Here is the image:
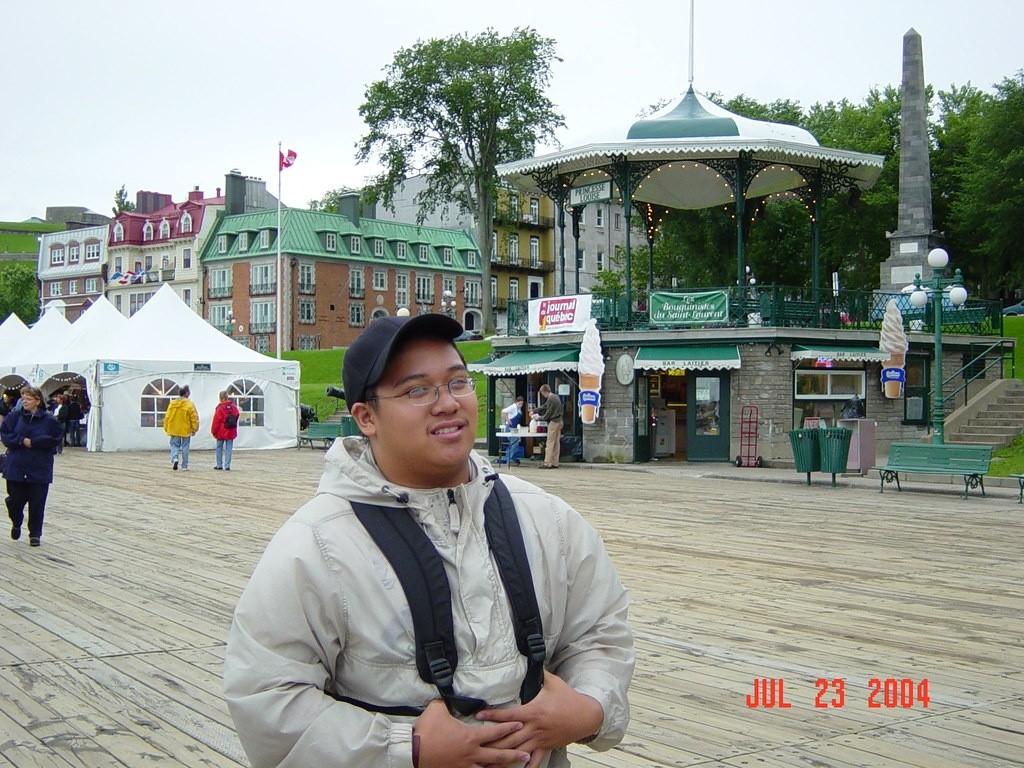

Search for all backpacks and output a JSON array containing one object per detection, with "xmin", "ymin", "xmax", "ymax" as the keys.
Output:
[{"xmin": 222, "ymin": 405, "xmax": 237, "ymax": 428}]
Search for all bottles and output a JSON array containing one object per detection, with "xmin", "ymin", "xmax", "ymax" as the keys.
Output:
[
  {"xmin": 529, "ymin": 419, "xmax": 537, "ymax": 433},
  {"xmin": 517, "ymin": 423, "xmax": 521, "ymax": 435}
]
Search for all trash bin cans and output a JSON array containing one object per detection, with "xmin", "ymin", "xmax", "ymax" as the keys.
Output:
[
  {"xmin": 340, "ymin": 415, "xmax": 360, "ymax": 436},
  {"xmin": 788, "ymin": 428, "xmax": 854, "ymax": 474}
]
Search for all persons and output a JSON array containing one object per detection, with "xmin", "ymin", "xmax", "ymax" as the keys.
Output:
[
  {"xmin": 670, "ymin": 385, "xmax": 681, "ymax": 402},
  {"xmin": 529, "ymin": 385, "xmax": 563, "ymax": 469},
  {"xmin": 0, "ymin": 388, "xmax": 30, "ymax": 440},
  {"xmin": 0, "ymin": 388, "xmax": 63, "ymax": 546},
  {"xmin": 502, "ymin": 396, "xmax": 524, "ymax": 466},
  {"xmin": 163, "ymin": 385, "xmax": 199, "ymax": 471},
  {"xmin": 47, "ymin": 391, "xmax": 82, "ymax": 454},
  {"xmin": 212, "ymin": 391, "xmax": 239, "ymax": 470},
  {"xmin": 223, "ymin": 314, "xmax": 636, "ymax": 768}
]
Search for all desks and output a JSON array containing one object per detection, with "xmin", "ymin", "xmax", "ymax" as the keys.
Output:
[{"xmin": 496, "ymin": 432, "xmax": 565, "ymax": 470}]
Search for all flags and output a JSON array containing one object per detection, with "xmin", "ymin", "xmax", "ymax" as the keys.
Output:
[
  {"xmin": 111, "ymin": 269, "xmax": 145, "ymax": 284},
  {"xmin": 280, "ymin": 149, "xmax": 297, "ymax": 171}
]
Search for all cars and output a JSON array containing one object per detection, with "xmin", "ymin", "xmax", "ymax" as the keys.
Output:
[{"xmin": 1002, "ymin": 299, "xmax": 1024, "ymax": 317}]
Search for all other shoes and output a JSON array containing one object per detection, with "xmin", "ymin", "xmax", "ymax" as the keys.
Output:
[
  {"xmin": 30, "ymin": 537, "xmax": 40, "ymax": 546},
  {"xmin": 552, "ymin": 466, "xmax": 558, "ymax": 469},
  {"xmin": 183, "ymin": 469, "xmax": 188, "ymax": 470},
  {"xmin": 173, "ymin": 460, "xmax": 179, "ymax": 470},
  {"xmin": 11, "ymin": 526, "xmax": 21, "ymax": 539},
  {"xmin": 214, "ymin": 467, "xmax": 222, "ymax": 470},
  {"xmin": 538, "ymin": 465, "xmax": 552, "ymax": 468},
  {"xmin": 226, "ymin": 468, "xmax": 230, "ymax": 470}
]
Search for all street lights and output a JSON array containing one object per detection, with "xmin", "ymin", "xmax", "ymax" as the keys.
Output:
[
  {"xmin": 909, "ymin": 247, "xmax": 968, "ymax": 445},
  {"xmin": 290, "ymin": 257, "xmax": 297, "ymax": 351},
  {"xmin": 225, "ymin": 310, "xmax": 236, "ymax": 336},
  {"xmin": 440, "ymin": 290, "xmax": 457, "ymax": 318}
]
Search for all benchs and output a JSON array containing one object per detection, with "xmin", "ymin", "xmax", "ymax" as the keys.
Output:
[
  {"xmin": 597, "ymin": 298, "xmax": 815, "ymax": 331},
  {"xmin": 873, "ymin": 305, "xmax": 986, "ymax": 335},
  {"xmin": 870, "ymin": 441, "xmax": 994, "ymax": 500},
  {"xmin": 298, "ymin": 421, "xmax": 342, "ymax": 452},
  {"xmin": 1009, "ymin": 471, "xmax": 1024, "ymax": 503}
]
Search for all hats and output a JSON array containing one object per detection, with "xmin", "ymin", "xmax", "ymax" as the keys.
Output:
[{"xmin": 342, "ymin": 313, "xmax": 464, "ymax": 413}]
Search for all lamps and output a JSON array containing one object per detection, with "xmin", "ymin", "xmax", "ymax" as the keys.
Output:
[
  {"xmin": 605, "ymin": 351, "xmax": 612, "ymax": 363},
  {"xmin": 765, "ymin": 343, "xmax": 772, "ymax": 357},
  {"xmin": 774, "ymin": 345, "xmax": 785, "ymax": 357}
]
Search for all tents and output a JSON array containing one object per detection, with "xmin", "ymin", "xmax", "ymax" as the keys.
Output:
[{"xmin": 0, "ymin": 283, "xmax": 301, "ymax": 452}]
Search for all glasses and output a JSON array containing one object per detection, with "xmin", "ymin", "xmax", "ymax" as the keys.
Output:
[{"xmin": 365, "ymin": 377, "xmax": 477, "ymax": 406}]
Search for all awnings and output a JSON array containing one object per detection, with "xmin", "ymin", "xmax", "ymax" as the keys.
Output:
[
  {"xmin": 484, "ymin": 348, "xmax": 579, "ymax": 394},
  {"xmin": 790, "ymin": 345, "xmax": 891, "ymax": 372},
  {"xmin": 467, "ymin": 357, "xmax": 492, "ymax": 373},
  {"xmin": 634, "ymin": 347, "xmax": 741, "ymax": 370}
]
